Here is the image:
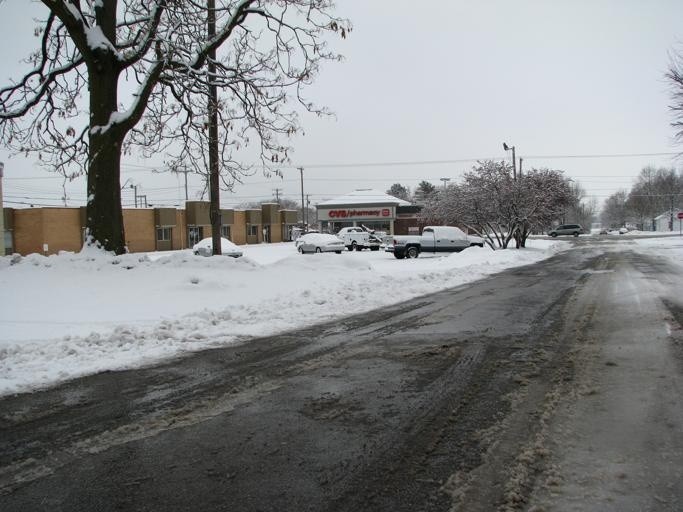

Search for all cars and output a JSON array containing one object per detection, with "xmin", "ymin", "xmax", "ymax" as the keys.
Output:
[
  {"xmin": 619, "ymin": 227, "xmax": 628, "ymax": 233},
  {"xmin": 294, "ymin": 233, "xmax": 345, "ymax": 254},
  {"xmin": 192, "ymin": 236, "xmax": 242, "ymax": 259}
]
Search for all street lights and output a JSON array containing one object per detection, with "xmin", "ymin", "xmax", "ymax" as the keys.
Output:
[
  {"xmin": 503, "ymin": 143, "xmax": 516, "ymax": 182},
  {"xmin": 440, "ymin": 178, "xmax": 450, "ymax": 188}
]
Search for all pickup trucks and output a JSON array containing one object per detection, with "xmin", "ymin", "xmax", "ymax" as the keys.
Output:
[
  {"xmin": 334, "ymin": 226, "xmax": 387, "ymax": 251},
  {"xmin": 384, "ymin": 225, "xmax": 485, "ymax": 258}
]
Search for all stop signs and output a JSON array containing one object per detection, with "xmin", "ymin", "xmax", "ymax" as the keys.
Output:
[{"xmin": 677, "ymin": 213, "xmax": 683, "ymax": 219}]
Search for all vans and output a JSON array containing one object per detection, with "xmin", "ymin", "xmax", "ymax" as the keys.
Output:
[{"xmin": 548, "ymin": 223, "xmax": 584, "ymax": 237}]
